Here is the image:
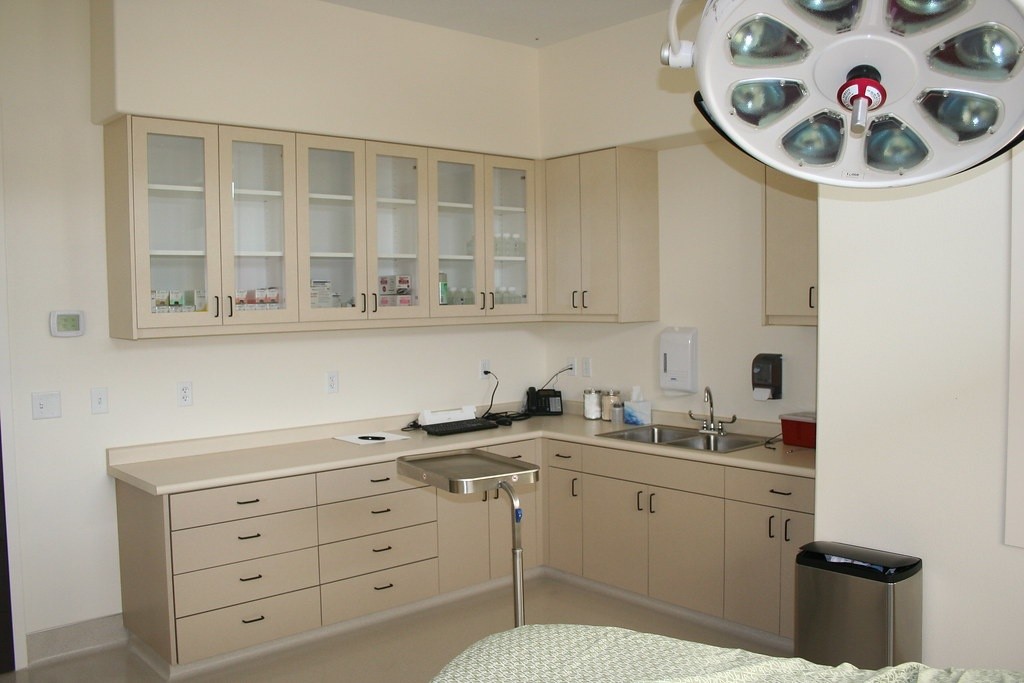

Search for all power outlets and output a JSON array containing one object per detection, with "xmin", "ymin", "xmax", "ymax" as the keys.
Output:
[
  {"xmin": 326, "ymin": 370, "xmax": 338, "ymax": 394},
  {"xmin": 479, "ymin": 358, "xmax": 491, "ymax": 380},
  {"xmin": 177, "ymin": 381, "xmax": 192, "ymax": 408},
  {"xmin": 566, "ymin": 356, "xmax": 576, "ymax": 377}
]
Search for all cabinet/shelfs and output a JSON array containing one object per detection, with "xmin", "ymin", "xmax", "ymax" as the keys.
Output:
[
  {"xmin": 426, "ymin": 147, "xmax": 543, "ymax": 326},
  {"xmin": 104, "ymin": 114, "xmax": 298, "ymax": 339},
  {"xmin": 546, "ymin": 143, "xmax": 660, "ymax": 325},
  {"xmin": 295, "ymin": 132, "xmax": 429, "ymax": 333},
  {"xmin": 114, "ymin": 437, "xmax": 815, "ymax": 679},
  {"xmin": 760, "ymin": 162, "xmax": 818, "ymax": 329}
]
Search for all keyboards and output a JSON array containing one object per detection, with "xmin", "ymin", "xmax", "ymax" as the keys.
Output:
[{"xmin": 421, "ymin": 418, "xmax": 498, "ymax": 436}]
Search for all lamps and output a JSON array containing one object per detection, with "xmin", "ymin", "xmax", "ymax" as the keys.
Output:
[{"xmin": 659, "ymin": 0, "xmax": 1024, "ymax": 189}]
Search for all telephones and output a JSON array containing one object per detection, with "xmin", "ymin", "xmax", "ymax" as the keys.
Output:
[{"xmin": 526, "ymin": 386, "xmax": 563, "ymax": 415}]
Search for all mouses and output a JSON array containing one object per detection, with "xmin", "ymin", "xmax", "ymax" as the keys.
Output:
[{"xmin": 496, "ymin": 418, "xmax": 512, "ymax": 426}]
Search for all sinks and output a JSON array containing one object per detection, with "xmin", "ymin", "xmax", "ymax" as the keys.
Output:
[
  {"xmin": 594, "ymin": 424, "xmax": 700, "ymax": 445},
  {"xmin": 666, "ymin": 434, "xmax": 782, "ymax": 454}
]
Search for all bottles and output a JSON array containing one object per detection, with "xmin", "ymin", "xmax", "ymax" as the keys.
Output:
[
  {"xmin": 447, "ymin": 287, "xmax": 522, "ymax": 306},
  {"xmin": 612, "ymin": 402, "xmax": 624, "ymax": 425},
  {"xmin": 466, "ymin": 233, "xmax": 523, "ymax": 257}
]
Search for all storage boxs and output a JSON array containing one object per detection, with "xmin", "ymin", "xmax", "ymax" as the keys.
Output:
[
  {"xmin": 150, "ymin": 290, "xmax": 208, "ymax": 313},
  {"xmin": 378, "ymin": 275, "xmax": 411, "ymax": 306},
  {"xmin": 235, "ymin": 288, "xmax": 281, "ymax": 311},
  {"xmin": 310, "ymin": 280, "xmax": 342, "ymax": 307}
]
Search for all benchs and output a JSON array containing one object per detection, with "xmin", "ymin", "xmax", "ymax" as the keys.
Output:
[{"xmin": 432, "ymin": 623, "xmax": 1024, "ymax": 683}]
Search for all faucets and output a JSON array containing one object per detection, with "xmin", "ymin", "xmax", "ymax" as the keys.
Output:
[{"xmin": 687, "ymin": 386, "xmax": 737, "ymax": 435}]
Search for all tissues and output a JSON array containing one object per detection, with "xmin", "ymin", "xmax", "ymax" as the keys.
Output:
[
  {"xmin": 750, "ymin": 353, "xmax": 783, "ymax": 402},
  {"xmin": 624, "ymin": 386, "xmax": 651, "ymax": 425}
]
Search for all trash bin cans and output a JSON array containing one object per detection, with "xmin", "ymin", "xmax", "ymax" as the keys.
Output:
[{"xmin": 796, "ymin": 542, "xmax": 922, "ymax": 670}]
